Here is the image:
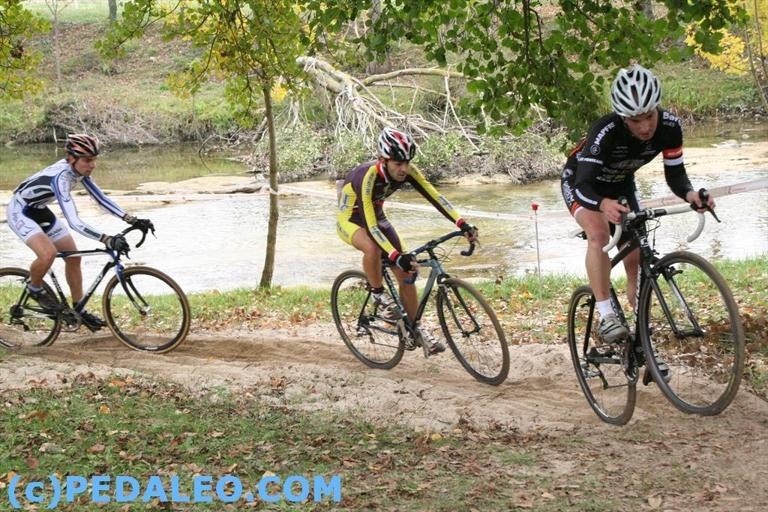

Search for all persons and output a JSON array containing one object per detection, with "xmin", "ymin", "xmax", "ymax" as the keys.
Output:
[
  {"xmin": 335, "ymin": 124, "xmax": 477, "ymax": 352},
  {"xmin": 7, "ymin": 135, "xmax": 155, "ymax": 328},
  {"xmin": 561, "ymin": 64, "xmax": 715, "ymax": 384}
]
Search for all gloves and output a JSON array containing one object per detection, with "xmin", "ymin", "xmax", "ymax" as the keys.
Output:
[
  {"xmin": 129, "ymin": 217, "xmax": 150, "ymax": 233},
  {"xmin": 105, "ymin": 234, "xmax": 130, "ymax": 251}
]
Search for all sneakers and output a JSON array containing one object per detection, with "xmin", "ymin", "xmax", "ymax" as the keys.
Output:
[
  {"xmin": 75, "ymin": 311, "xmax": 103, "ymax": 328},
  {"xmin": 408, "ymin": 327, "xmax": 445, "ymax": 352},
  {"xmin": 26, "ymin": 283, "xmax": 58, "ymax": 309},
  {"xmin": 371, "ymin": 290, "xmax": 402, "ymax": 320},
  {"xmin": 600, "ymin": 313, "xmax": 628, "ymax": 342},
  {"xmin": 636, "ymin": 339, "xmax": 672, "ymax": 383}
]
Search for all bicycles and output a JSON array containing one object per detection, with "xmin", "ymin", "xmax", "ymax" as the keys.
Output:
[
  {"xmin": 331, "ymin": 229, "xmax": 510, "ymax": 385},
  {"xmin": 566, "ymin": 188, "xmax": 745, "ymax": 426},
  {"xmin": 0, "ymin": 225, "xmax": 191, "ymax": 355}
]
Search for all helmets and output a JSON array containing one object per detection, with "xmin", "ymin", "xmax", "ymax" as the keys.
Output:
[
  {"xmin": 609, "ymin": 64, "xmax": 662, "ymax": 117},
  {"xmin": 379, "ymin": 126, "xmax": 416, "ymax": 161},
  {"xmin": 65, "ymin": 134, "xmax": 100, "ymax": 157}
]
